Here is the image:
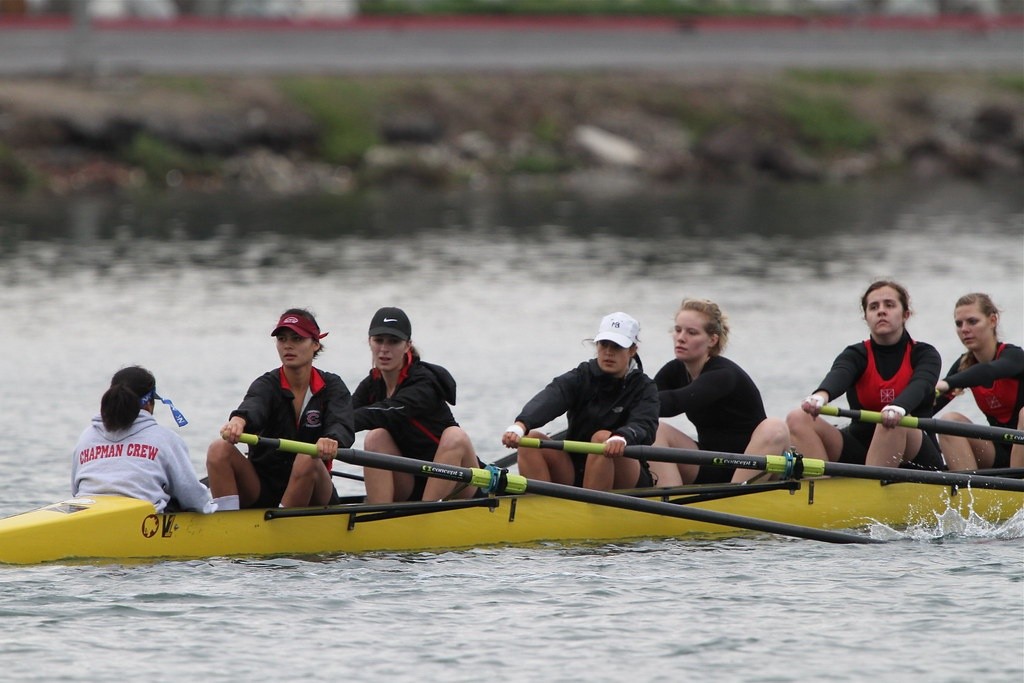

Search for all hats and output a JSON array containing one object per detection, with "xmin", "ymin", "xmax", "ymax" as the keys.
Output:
[
  {"xmin": 591, "ymin": 310, "xmax": 642, "ymax": 348},
  {"xmin": 368, "ymin": 307, "xmax": 414, "ymax": 343},
  {"xmin": 270, "ymin": 313, "xmax": 322, "ymax": 345}
]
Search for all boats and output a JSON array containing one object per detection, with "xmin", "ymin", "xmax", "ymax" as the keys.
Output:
[{"xmin": 2, "ymin": 465, "xmax": 1024, "ymax": 565}]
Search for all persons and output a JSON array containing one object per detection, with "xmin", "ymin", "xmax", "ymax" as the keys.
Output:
[
  {"xmin": 351, "ymin": 307, "xmax": 495, "ymax": 504},
  {"xmin": 500, "ymin": 311, "xmax": 661, "ymax": 494},
  {"xmin": 786, "ymin": 280, "xmax": 943, "ymax": 480},
  {"xmin": 648, "ymin": 301, "xmax": 789, "ymax": 491},
  {"xmin": 71, "ymin": 367, "xmax": 216, "ymax": 516},
  {"xmin": 206, "ymin": 309, "xmax": 356, "ymax": 513},
  {"xmin": 934, "ymin": 292, "xmax": 1024, "ymax": 472}
]
